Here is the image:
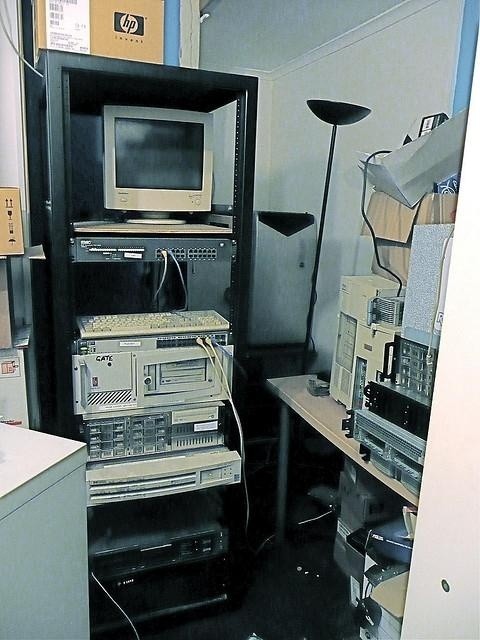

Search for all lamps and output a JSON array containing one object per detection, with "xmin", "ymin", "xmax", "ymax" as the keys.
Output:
[{"xmin": 258, "ymin": 99, "xmax": 371, "ymax": 500}]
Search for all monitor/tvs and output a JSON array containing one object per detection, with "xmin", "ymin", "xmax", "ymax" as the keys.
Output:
[{"xmin": 104, "ymin": 106, "xmax": 215, "ymax": 225}]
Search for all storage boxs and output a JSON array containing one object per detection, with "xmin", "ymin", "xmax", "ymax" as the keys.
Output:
[
  {"xmin": 0, "ymin": 186, "xmax": 46, "ymax": 429},
  {"xmin": 361, "ymin": 190, "xmax": 457, "ymax": 286},
  {"xmin": 30, "ymin": 1, "xmax": 166, "ymax": 66}
]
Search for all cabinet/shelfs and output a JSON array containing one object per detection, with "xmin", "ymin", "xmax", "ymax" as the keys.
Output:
[{"xmin": 22, "ymin": 53, "xmax": 259, "ymax": 609}]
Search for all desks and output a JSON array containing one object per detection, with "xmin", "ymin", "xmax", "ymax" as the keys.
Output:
[
  {"xmin": 0, "ymin": 426, "xmax": 94, "ymax": 640},
  {"xmin": 267, "ymin": 373, "xmax": 420, "ymax": 544}
]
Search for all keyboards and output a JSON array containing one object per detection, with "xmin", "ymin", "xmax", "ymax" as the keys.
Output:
[{"xmin": 77, "ymin": 309, "xmax": 230, "ymax": 339}]
